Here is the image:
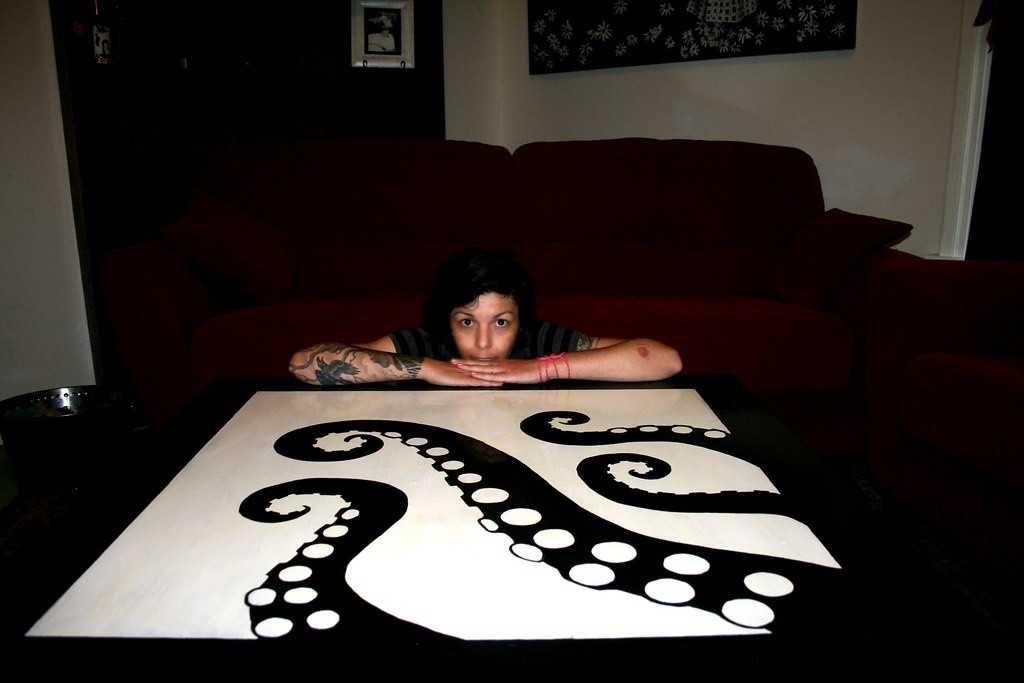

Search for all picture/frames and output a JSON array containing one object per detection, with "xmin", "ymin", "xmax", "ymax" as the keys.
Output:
[{"xmin": 351, "ymin": 1, "xmax": 416, "ymax": 69}]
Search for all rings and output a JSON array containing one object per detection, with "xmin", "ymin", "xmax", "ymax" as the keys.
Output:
[
  {"xmin": 488, "ymin": 360, "xmax": 496, "ymax": 366},
  {"xmin": 490, "ymin": 366, "xmax": 495, "ymax": 373}
]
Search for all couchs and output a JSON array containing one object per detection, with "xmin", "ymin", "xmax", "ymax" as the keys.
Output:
[{"xmin": 98, "ymin": 140, "xmax": 1024, "ymax": 587}]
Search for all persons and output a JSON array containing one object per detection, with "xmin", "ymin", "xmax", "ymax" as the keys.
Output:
[
  {"xmin": 288, "ymin": 248, "xmax": 684, "ymax": 387},
  {"xmin": 93, "ymin": 26, "xmax": 111, "ymax": 56},
  {"xmin": 367, "ymin": 13, "xmax": 397, "ymax": 54}
]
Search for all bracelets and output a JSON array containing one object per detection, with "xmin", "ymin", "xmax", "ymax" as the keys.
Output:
[{"xmin": 537, "ymin": 352, "xmax": 571, "ymax": 382}]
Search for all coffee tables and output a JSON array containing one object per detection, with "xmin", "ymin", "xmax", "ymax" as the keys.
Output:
[{"xmin": 0, "ymin": 372, "xmax": 1024, "ymax": 683}]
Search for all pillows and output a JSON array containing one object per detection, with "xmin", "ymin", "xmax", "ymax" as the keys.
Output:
[
  {"xmin": 760, "ymin": 209, "xmax": 913, "ymax": 304},
  {"xmin": 160, "ymin": 191, "xmax": 300, "ymax": 308}
]
